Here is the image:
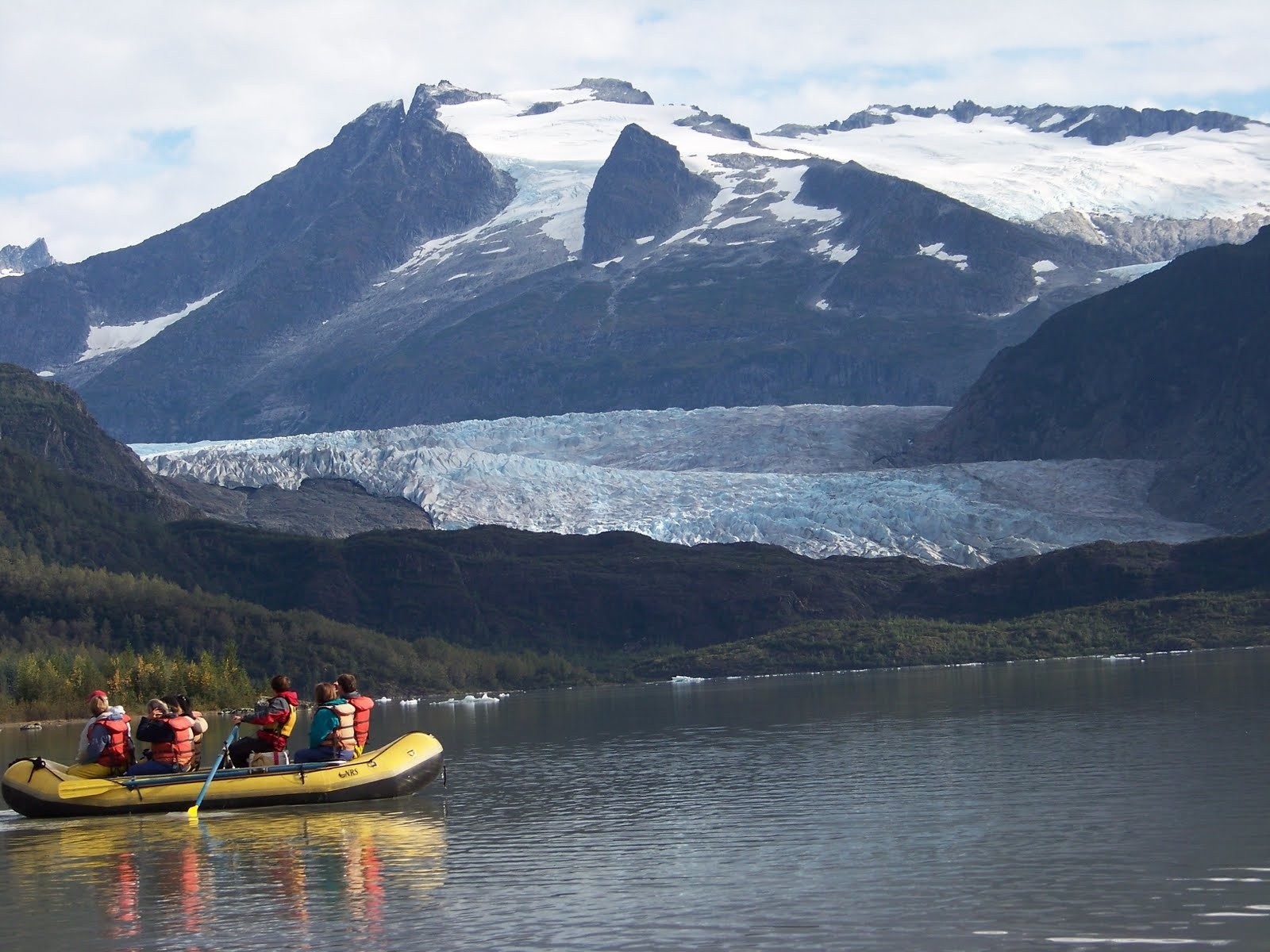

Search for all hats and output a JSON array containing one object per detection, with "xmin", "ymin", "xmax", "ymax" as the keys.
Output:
[{"xmin": 83, "ymin": 690, "xmax": 108, "ymax": 705}]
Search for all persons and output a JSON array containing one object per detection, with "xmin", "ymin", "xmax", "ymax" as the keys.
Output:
[
  {"xmin": 226, "ymin": 675, "xmax": 298, "ymax": 768},
  {"xmin": 294, "ymin": 684, "xmax": 356, "ymax": 763},
  {"xmin": 338, "ymin": 674, "xmax": 374, "ymax": 758},
  {"xmin": 66, "ymin": 690, "xmax": 132, "ymax": 779},
  {"xmin": 127, "ymin": 695, "xmax": 208, "ymax": 775}
]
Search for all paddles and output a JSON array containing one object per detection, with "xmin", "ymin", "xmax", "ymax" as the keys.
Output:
[
  {"xmin": 187, "ymin": 721, "xmax": 242, "ymax": 818},
  {"xmin": 57, "ymin": 761, "xmax": 341, "ymax": 800}
]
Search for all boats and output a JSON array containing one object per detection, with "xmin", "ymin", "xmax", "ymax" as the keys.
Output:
[{"xmin": 1, "ymin": 731, "xmax": 445, "ymax": 819}]
